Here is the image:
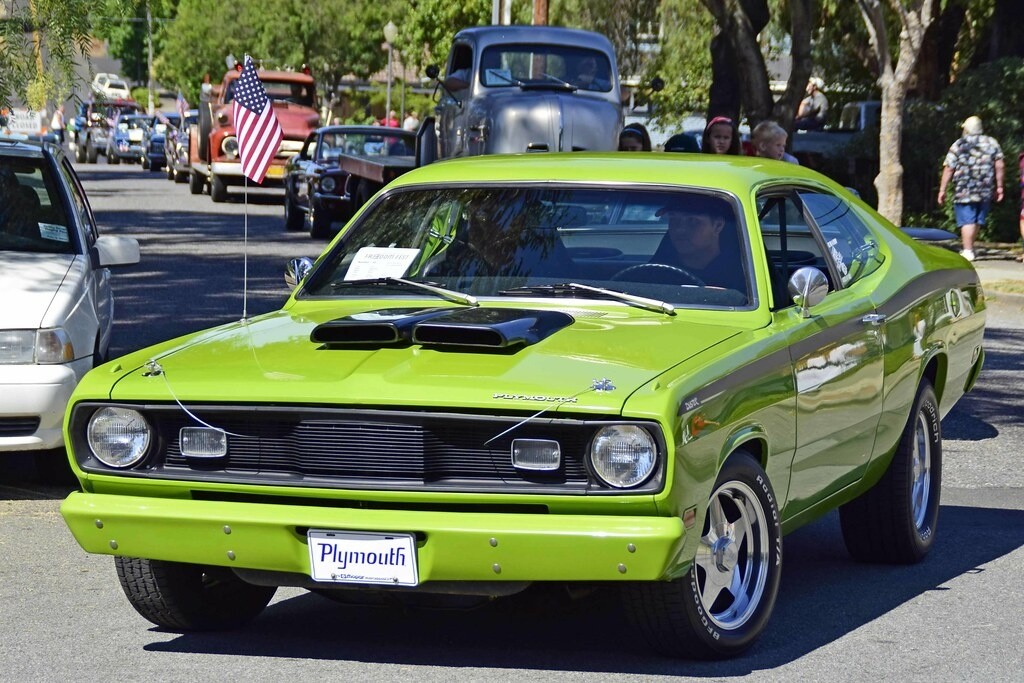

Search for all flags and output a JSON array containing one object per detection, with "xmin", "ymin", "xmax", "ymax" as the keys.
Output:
[
  {"xmin": 175, "ymin": 90, "xmax": 189, "ymax": 117},
  {"xmin": 154, "ymin": 112, "xmax": 170, "ymax": 125},
  {"xmin": 232, "ymin": 54, "xmax": 283, "ymax": 183}
]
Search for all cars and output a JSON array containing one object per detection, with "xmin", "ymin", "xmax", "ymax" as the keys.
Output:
[
  {"xmin": 142, "ymin": 112, "xmax": 182, "ymax": 173},
  {"xmin": 90, "ymin": 72, "xmax": 118, "ymax": 94},
  {"xmin": 1, "ymin": 137, "xmax": 141, "ymax": 484},
  {"xmin": 165, "ymin": 110, "xmax": 198, "ymax": 184},
  {"xmin": 61, "ymin": 151, "xmax": 987, "ymax": 662},
  {"xmin": 106, "ymin": 115, "xmax": 154, "ymax": 166},
  {"xmin": 282, "ymin": 124, "xmax": 417, "ymax": 240},
  {"xmin": 74, "ymin": 99, "xmax": 144, "ymax": 164},
  {"xmin": 101, "ymin": 79, "xmax": 130, "ymax": 99}
]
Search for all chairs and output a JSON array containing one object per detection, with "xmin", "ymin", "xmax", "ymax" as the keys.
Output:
[
  {"xmin": 0, "ymin": 163, "xmax": 42, "ymax": 236},
  {"xmin": 526, "ymin": 199, "xmax": 784, "ymax": 309}
]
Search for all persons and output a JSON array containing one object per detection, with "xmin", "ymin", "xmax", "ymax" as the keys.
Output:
[
  {"xmin": 654, "ymin": 192, "xmax": 777, "ymax": 318},
  {"xmin": 617, "ymin": 122, "xmax": 656, "ymax": 153},
  {"xmin": 435, "ymin": 194, "xmax": 573, "ymax": 282},
  {"xmin": 1014, "ymin": 147, "xmax": 1024, "ymax": 264},
  {"xmin": 700, "ymin": 117, "xmax": 740, "ymax": 158},
  {"xmin": 658, "ymin": 129, "xmax": 698, "ymax": 153},
  {"xmin": 749, "ymin": 116, "xmax": 792, "ymax": 167},
  {"xmin": 795, "ymin": 71, "xmax": 831, "ymax": 134},
  {"xmin": 402, "ymin": 111, "xmax": 411, "ymax": 131},
  {"xmin": 937, "ymin": 113, "xmax": 1007, "ymax": 266},
  {"xmin": 408, "ymin": 109, "xmax": 419, "ymax": 132},
  {"xmin": 380, "ymin": 110, "xmax": 400, "ymax": 146},
  {"xmin": 48, "ymin": 102, "xmax": 71, "ymax": 144}
]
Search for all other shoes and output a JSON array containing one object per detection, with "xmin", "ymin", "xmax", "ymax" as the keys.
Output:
[{"xmin": 961, "ymin": 249, "xmax": 976, "ymax": 261}]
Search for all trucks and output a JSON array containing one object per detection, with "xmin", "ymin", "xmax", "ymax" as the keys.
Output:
[
  {"xmin": 188, "ymin": 71, "xmax": 325, "ymax": 203},
  {"xmin": 684, "ymin": 102, "xmax": 943, "ymax": 203},
  {"xmin": 337, "ymin": 24, "xmax": 664, "ymax": 222}
]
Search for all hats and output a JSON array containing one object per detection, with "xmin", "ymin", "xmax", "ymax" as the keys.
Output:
[
  {"xmin": 655, "ymin": 193, "xmax": 723, "ymax": 218},
  {"xmin": 960, "ymin": 116, "xmax": 983, "ymax": 131}
]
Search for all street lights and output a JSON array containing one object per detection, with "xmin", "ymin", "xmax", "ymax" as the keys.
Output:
[{"xmin": 384, "ymin": 20, "xmax": 398, "ymax": 128}]
